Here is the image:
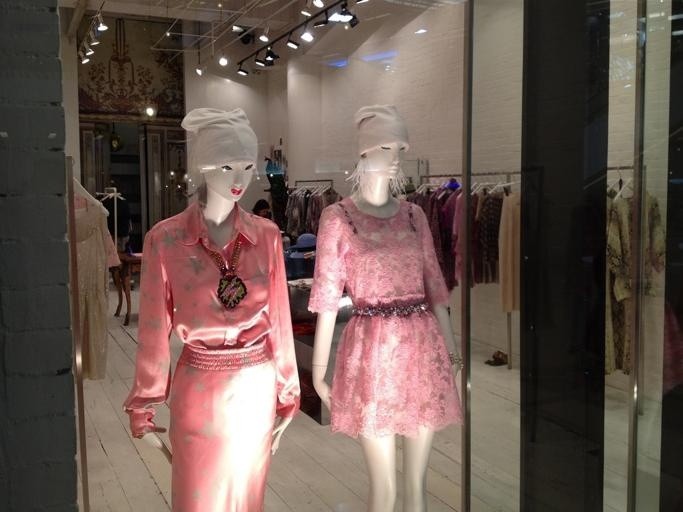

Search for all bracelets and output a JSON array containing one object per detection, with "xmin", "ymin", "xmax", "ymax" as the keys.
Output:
[{"xmin": 449, "ymin": 351, "xmax": 465, "ymax": 369}]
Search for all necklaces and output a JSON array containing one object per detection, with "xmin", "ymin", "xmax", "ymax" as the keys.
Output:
[{"xmin": 199, "ymin": 234, "xmax": 247, "ymax": 308}]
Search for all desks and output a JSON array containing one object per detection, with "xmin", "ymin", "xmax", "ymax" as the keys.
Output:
[{"xmin": 109, "ymin": 252, "xmax": 143, "ymax": 326}]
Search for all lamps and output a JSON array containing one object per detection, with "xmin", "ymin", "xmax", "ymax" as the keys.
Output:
[
  {"xmin": 78, "ymin": 0, "xmax": 109, "ymax": 64},
  {"xmin": 110, "ymin": 122, "xmax": 120, "ymax": 148},
  {"xmin": 194, "ymin": 0, "xmax": 369, "ymax": 77},
  {"xmin": 92, "ymin": 122, "xmax": 105, "ymax": 140}
]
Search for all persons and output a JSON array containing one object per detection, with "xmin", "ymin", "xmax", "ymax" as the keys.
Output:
[
  {"xmin": 250, "ymin": 198, "xmax": 270, "ymax": 219},
  {"xmin": 311, "ymin": 102, "xmax": 468, "ymax": 512},
  {"xmin": 122, "ymin": 106, "xmax": 302, "ymax": 512}
]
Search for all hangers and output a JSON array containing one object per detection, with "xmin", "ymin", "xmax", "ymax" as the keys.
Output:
[
  {"xmin": 614, "ymin": 165, "xmax": 634, "ymax": 199},
  {"xmin": 606, "ymin": 165, "xmax": 627, "ymax": 195},
  {"xmin": 289, "ymin": 184, "xmax": 344, "ymax": 199},
  {"xmin": 416, "ymin": 169, "xmax": 521, "ymax": 201},
  {"xmin": 66, "ymin": 158, "xmax": 110, "ymax": 216}
]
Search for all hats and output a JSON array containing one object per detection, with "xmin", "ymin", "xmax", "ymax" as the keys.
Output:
[
  {"xmin": 182, "ymin": 107, "xmax": 258, "ymax": 168},
  {"xmin": 355, "ymin": 104, "xmax": 410, "ymax": 158},
  {"xmin": 286, "ymin": 233, "xmax": 317, "ymax": 249}
]
